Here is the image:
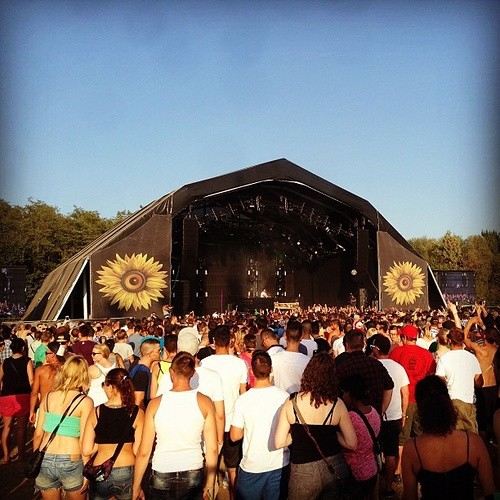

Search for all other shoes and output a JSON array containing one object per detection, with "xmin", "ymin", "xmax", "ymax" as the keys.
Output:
[{"xmin": 0, "ymin": 457, "xmax": 25, "ymax": 465}]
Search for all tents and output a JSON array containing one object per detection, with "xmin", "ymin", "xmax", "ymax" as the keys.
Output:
[{"xmin": 18, "ymin": 157, "xmax": 448, "ymax": 318}]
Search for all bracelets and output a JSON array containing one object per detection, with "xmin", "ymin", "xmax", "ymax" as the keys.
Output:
[
  {"xmin": 452, "ymin": 313, "xmax": 458, "ymax": 316},
  {"xmin": 402, "ymin": 412, "xmax": 409, "ymax": 419}
]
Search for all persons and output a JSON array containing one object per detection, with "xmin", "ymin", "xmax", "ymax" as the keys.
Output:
[{"xmin": 0, "ymin": 288, "xmax": 500, "ymax": 500}]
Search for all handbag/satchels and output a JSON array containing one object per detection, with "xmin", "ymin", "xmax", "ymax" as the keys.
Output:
[
  {"xmin": 217, "ymin": 438, "xmax": 242, "ymax": 469},
  {"xmin": 24, "ymin": 449, "xmax": 45, "ymax": 478},
  {"xmin": 374, "ymin": 444, "xmax": 385, "ymax": 471},
  {"xmin": 83, "ymin": 450, "xmax": 115, "ymax": 483},
  {"xmin": 216, "ymin": 476, "xmax": 237, "ymax": 500}
]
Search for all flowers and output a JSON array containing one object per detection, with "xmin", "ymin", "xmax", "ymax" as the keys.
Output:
[
  {"xmin": 94, "ymin": 252, "xmax": 168, "ymax": 312},
  {"xmin": 382, "ymin": 260, "xmax": 426, "ymax": 306}
]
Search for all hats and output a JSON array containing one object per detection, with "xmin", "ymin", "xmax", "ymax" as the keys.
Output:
[
  {"xmin": 447, "ymin": 328, "xmax": 464, "ymax": 344},
  {"xmin": 397, "ymin": 324, "xmax": 418, "ymax": 339}
]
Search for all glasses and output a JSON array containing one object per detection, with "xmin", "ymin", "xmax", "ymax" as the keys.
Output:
[
  {"xmin": 45, "ymin": 352, "xmax": 53, "ymax": 355},
  {"xmin": 92, "ymin": 352, "xmax": 101, "ymax": 356}
]
